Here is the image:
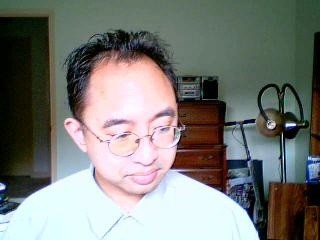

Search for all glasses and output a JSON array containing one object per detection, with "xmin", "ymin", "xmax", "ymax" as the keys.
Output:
[{"xmin": 75, "ymin": 114, "xmax": 185, "ymax": 156}]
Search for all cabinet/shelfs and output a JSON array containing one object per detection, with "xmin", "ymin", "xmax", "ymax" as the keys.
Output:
[{"xmin": 171, "ymin": 100, "xmax": 227, "ymax": 195}]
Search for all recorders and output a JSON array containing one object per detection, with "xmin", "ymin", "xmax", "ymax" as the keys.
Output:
[{"xmin": 173, "ymin": 75, "xmax": 219, "ymax": 102}]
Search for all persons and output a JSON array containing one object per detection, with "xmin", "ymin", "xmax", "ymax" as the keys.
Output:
[{"xmin": 1, "ymin": 28, "xmax": 260, "ymax": 240}]
